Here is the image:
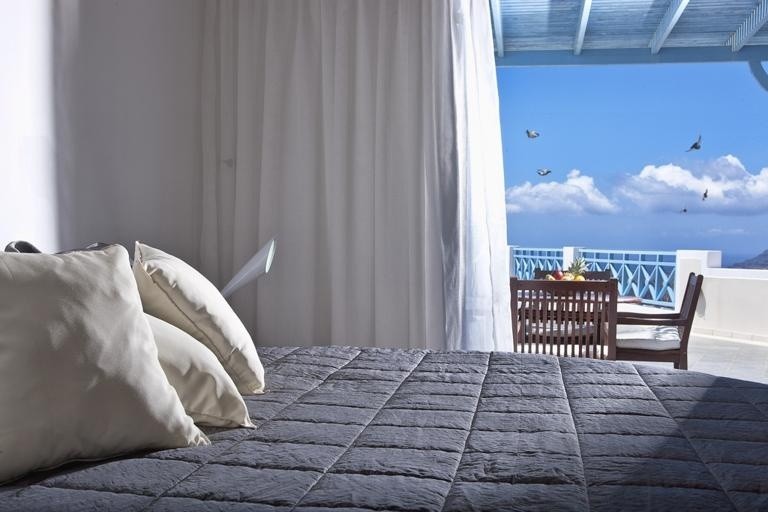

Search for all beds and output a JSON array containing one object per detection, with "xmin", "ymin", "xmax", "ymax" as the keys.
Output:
[{"xmin": 0, "ymin": 344, "xmax": 768, "ymax": 512}]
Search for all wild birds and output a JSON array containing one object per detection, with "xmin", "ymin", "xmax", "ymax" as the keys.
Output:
[
  {"xmin": 679, "ymin": 208, "xmax": 687, "ymax": 213},
  {"xmin": 685, "ymin": 135, "xmax": 701, "ymax": 152},
  {"xmin": 526, "ymin": 129, "xmax": 539, "ymax": 138},
  {"xmin": 702, "ymin": 189, "xmax": 708, "ymax": 200},
  {"xmin": 536, "ymin": 167, "xmax": 551, "ymax": 176}
]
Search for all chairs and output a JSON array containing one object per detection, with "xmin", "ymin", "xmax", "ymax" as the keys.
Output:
[{"xmin": 509, "ymin": 268, "xmax": 704, "ymax": 369}]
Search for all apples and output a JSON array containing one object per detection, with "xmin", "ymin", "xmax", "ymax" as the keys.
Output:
[{"xmin": 545, "ymin": 271, "xmax": 585, "ymax": 281}]
